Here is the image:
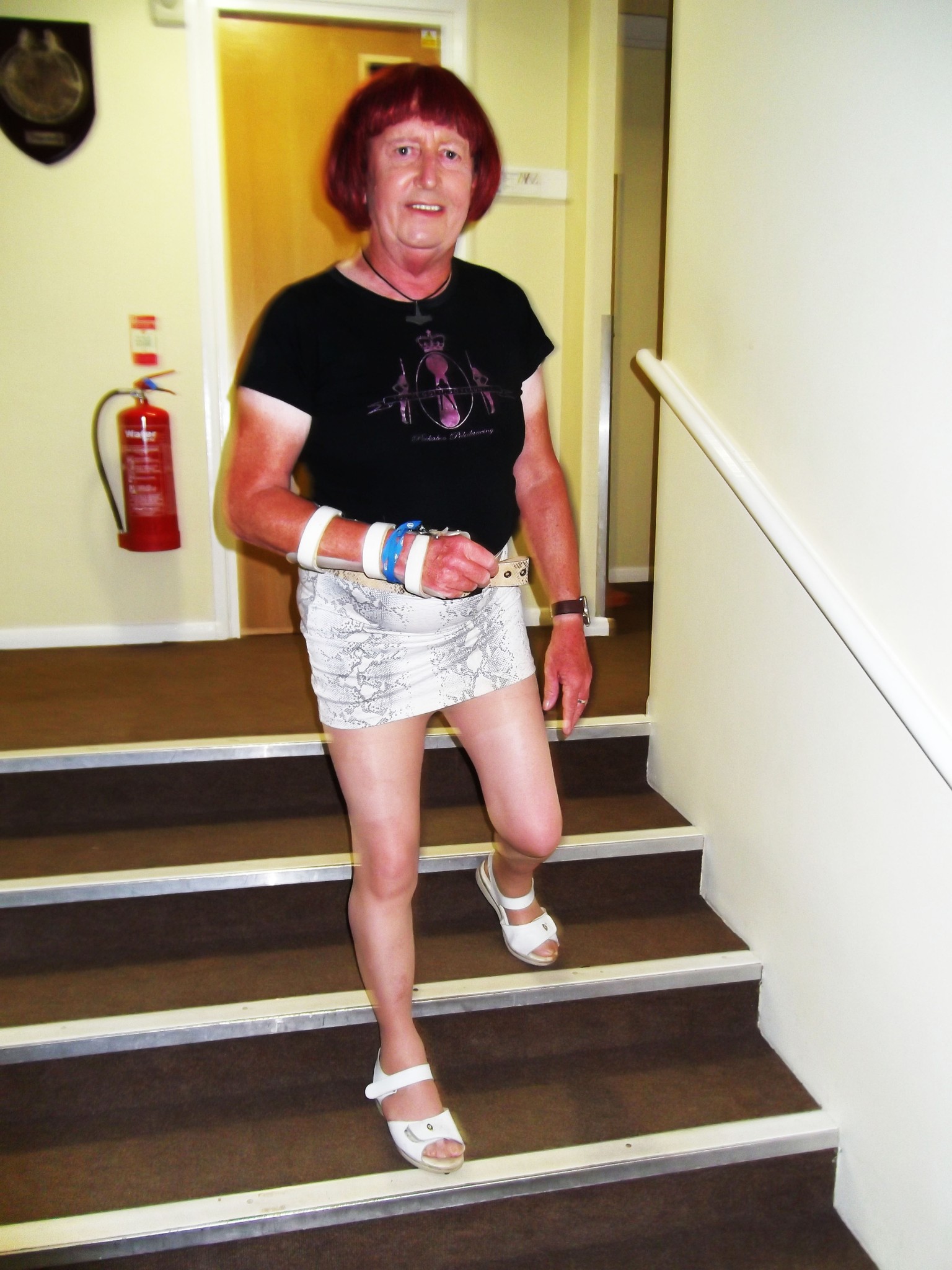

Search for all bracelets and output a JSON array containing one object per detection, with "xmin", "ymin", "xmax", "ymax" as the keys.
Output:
[
  {"xmin": 362, "ymin": 521, "xmax": 471, "ymax": 599},
  {"xmin": 297, "ymin": 505, "xmax": 342, "ymax": 574}
]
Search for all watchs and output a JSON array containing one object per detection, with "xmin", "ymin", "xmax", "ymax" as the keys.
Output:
[{"xmin": 550, "ymin": 595, "xmax": 591, "ymax": 627}]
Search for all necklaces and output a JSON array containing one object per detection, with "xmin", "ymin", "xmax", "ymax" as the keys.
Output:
[{"xmin": 361, "ymin": 247, "xmax": 451, "ymax": 325}]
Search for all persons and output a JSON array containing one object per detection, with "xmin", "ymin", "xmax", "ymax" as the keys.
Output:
[{"xmin": 221, "ymin": 57, "xmax": 593, "ymax": 1174}]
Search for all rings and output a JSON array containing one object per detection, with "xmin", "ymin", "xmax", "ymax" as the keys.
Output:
[{"xmin": 578, "ymin": 699, "xmax": 588, "ymax": 706}]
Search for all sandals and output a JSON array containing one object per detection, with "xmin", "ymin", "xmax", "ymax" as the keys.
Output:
[
  {"xmin": 474, "ymin": 853, "xmax": 560, "ymax": 966},
  {"xmin": 365, "ymin": 1046, "xmax": 466, "ymax": 1172}
]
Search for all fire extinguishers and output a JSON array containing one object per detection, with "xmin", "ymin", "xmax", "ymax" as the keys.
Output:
[{"xmin": 91, "ymin": 368, "xmax": 181, "ymax": 552}]
